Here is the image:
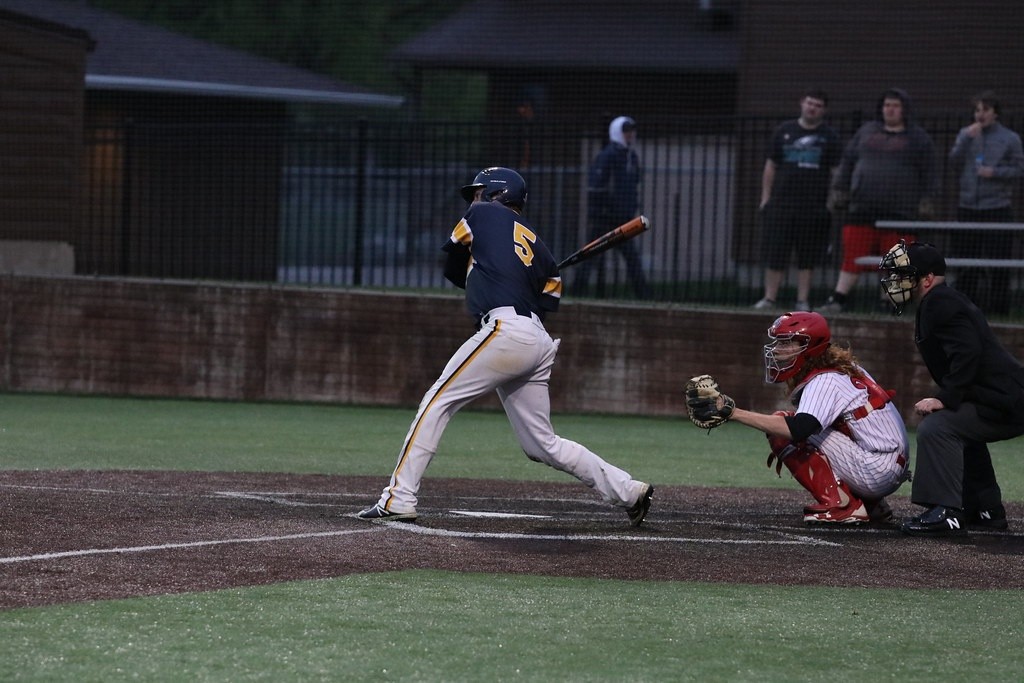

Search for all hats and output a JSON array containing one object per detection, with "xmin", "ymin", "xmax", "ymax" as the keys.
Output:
[{"xmin": 906, "ymin": 240, "xmax": 946, "ymax": 277}]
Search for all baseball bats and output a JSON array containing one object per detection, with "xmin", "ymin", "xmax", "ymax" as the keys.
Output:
[{"xmin": 557, "ymin": 215, "xmax": 650, "ymax": 273}]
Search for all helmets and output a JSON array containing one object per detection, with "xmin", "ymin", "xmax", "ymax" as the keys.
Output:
[
  {"xmin": 461, "ymin": 167, "xmax": 526, "ymax": 210},
  {"xmin": 764, "ymin": 311, "xmax": 831, "ymax": 383}
]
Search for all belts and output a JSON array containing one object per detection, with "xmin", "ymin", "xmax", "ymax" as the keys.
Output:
[
  {"xmin": 475, "ymin": 306, "xmax": 531, "ymax": 330},
  {"xmin": 897, "ymin": 456, "xmax": 905, "ymax": 469}
]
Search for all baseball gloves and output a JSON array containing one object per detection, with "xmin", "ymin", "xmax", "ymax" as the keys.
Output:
[{"xmin": 684, "ymin": 374, "xmax": 736, "ymax": 430}]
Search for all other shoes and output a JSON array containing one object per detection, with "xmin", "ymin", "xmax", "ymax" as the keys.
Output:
[
  {"xmin": 813, "ymin": 294, "xmax": 853, "ymax": 313},
  {"xmin": 752, "ymin": 298, "xmax": 777, "ymax": 312},
  {"xmin": 794, "ymin": 302, "xmax": 811, "ymax": 311}
]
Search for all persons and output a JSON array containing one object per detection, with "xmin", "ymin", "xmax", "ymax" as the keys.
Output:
[
  {"xmin": 684, "ymin": 310, "xmax": 912, "ymax": 522},
  {"xmin": 753, "ymin": 89, "xmax": 844, "ymax": 310},
  {"xmin": 822, "ymin": 89, "xmax": 941, "ymax": 309},
  {"xmin": 879, "ymin": 237, "xmax": 1024, "ymax": 536},
  {"xmin": 359, "ymin": 166, "xmax": 653, "ymax": 526},
  {"xmin": 948, "ymin": 92, "xmax": 1024, "ymax": 318},
  {"xmin": 576, "ymin": 117, "xmax": 647, "ymax": 298}
]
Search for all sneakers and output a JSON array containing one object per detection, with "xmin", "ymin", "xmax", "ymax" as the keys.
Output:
[
  {"xmin": 626, "ymin": 484, "xmax": 655, "ymax": 527},
  {"xmin": 964, "ymin": 504, "xmax": 1008, "ymax": 529},
  {"xmin": 357, "ymin": 503, "xmax": 417, "ymax": 521},
  {"xmin": 866, "ymin": 497, "xmax": 893, "ymax": 521},
  {"xmin": 804, "ymin": 500, "xmax": 870, "ymax": 526},
  {"xmin": 900, "ymin": 505, "xmax": 967, "ymax": 536}
]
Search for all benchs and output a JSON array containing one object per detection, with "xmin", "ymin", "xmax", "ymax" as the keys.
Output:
[{"xmin": 855, "ymin": 220, "xmax": 1024, "ymax": 314}]
